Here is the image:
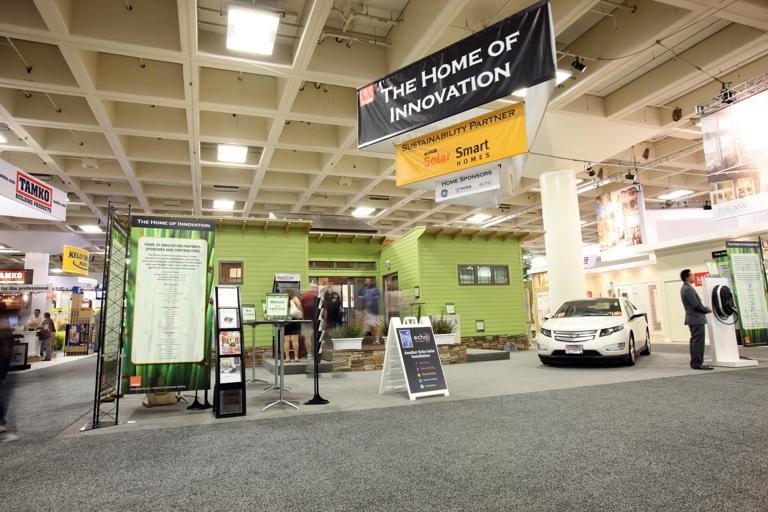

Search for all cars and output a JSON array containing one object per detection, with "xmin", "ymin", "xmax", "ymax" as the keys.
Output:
[{"xmin": 535, "ymin": 296, "xmax": 652, "ymax": 366}]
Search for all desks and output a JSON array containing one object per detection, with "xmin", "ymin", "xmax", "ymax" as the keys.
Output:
[{"xmin": 242, "ymin": 320, "xmax": 313, "ymax": 411}]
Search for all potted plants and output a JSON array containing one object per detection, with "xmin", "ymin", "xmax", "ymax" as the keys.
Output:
[
  {"xmin": 431, "ymin": 314, "xmax": 456, "ymax": 345},
  {"xmin": 375, "ymin": 319, "xmax": 389, "ymax": 348},
  {"xmin": 329, "ymin": 321, "xmax": 364, "ymax": 351}
]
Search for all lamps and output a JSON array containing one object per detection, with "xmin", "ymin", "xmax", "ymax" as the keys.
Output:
[
  {"xmin": 672, "ymin": 109, "xmax": 681, "ymax": 122},
  {"xmin": 642, "ymin": 149, "xmax": 649, "ymax": 160},
  {"xmin": 587, "ymin": 166, "xmax": 595, "ymax": 176},
  {"xmin": 625, "ymin": 172, "xmax": 636, "ymax": 180},
  {"xmin": 225, "ymin": 4, "xmax": 280, "ymax": 56},
  {"xmin": 571, "ymin": 57, "xmax": 586, "ymax": 73}
]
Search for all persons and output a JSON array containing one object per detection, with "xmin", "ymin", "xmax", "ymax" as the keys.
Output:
[
  {"xmin": 283, "ymin": 288, "xmax": 304, "ymax": 363},
  {"xmin": 680, "ymin": 269, "xmax": 713, "ymax": 369},
  {"xmin": 0, "ymin": 303, "xmax": 55, "ymax": 443},
  {"xmin": 358, "ymin": 278, "xmax": 381, "ymax": 315}
]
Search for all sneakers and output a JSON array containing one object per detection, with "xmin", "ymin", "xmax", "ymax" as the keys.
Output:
[{"xmin": 40, "ymin": 353, "xmax": 51, "ymax": 361}]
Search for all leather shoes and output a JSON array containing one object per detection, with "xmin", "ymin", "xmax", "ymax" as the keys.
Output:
[{"xmin": 702, "ymin": 365, "xmax": 714, "ymax": 370}]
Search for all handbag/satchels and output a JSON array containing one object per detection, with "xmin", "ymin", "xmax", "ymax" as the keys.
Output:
[
  {"xmin": 36, "ymin": 329, "xmax": 49, "ymax": 341},
  {"xmin": 289, "ymin": 299, "xmax": 304, "ymax": 319}
]
